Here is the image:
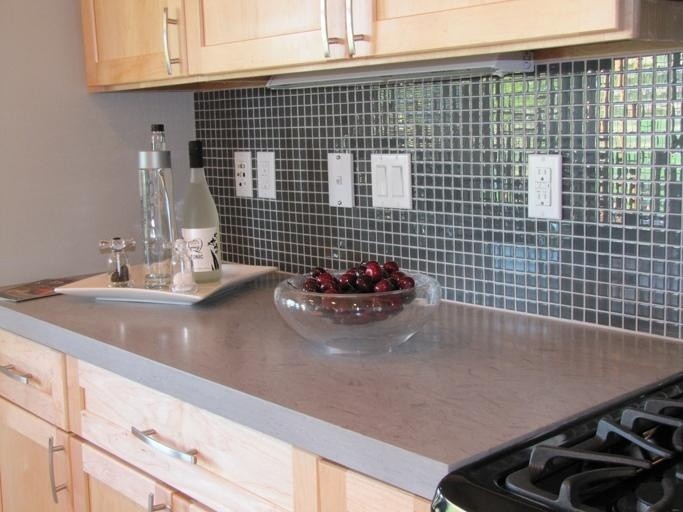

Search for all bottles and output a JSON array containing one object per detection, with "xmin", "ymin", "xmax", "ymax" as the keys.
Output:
[
  {"xmin": 107, "ymin": 237, "xmax": 134, "ymax": 289},
  {"xmin": 136, "ymin": 123, "xmax": 223, "ymax": 290}
]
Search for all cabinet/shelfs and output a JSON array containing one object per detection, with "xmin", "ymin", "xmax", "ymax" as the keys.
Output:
[
  {"xmin": 73, "ymin": 356, "xmax": 319, "ymax": 512},
  {"xmin": 0, "ymin": 327, "xmax": 73, "ymax": 512},
  {"xmin": 80, "ymin": 0, "xmax": 190, "ymax": 93},
  {"xmin": 317, "ymin": 458, "xmax": 435, "ymax": 512},
  {"xmin": 190, "ymin": 0, "xmax": 682, "ymax": 92}
]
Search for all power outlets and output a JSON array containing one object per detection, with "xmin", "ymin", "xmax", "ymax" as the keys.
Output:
[
  {"xmin": 528, "ymin": 153, "xmax": 563, "ymax": 219},
  {"xmin": 234, "ymin": 150, "xmax": 253, "ymax": 198}
]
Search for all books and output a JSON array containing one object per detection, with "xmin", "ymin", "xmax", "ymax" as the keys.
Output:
[{"xmin": 0, "ymin": 278, "xmax": 74, "ymax": 302}]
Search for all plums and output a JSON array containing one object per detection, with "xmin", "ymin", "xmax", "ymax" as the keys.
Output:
[{"xmin": 301, "ymin": 261, "xmax": 417, "ymax": 327}]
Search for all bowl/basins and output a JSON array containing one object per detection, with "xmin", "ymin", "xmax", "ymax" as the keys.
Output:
[{"xmin": 272, "ymin": 268, "xmax": 441, "ymax": 361}]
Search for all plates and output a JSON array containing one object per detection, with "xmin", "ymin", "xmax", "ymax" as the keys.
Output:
[{"xmin": 53, "ymin": 261, "xmax": 278, "ymax": 307}]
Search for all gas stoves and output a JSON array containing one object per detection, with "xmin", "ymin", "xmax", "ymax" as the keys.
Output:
[{"xmin": 432, "ymin": 370, "xmax": 683, "ymax": 511}]
